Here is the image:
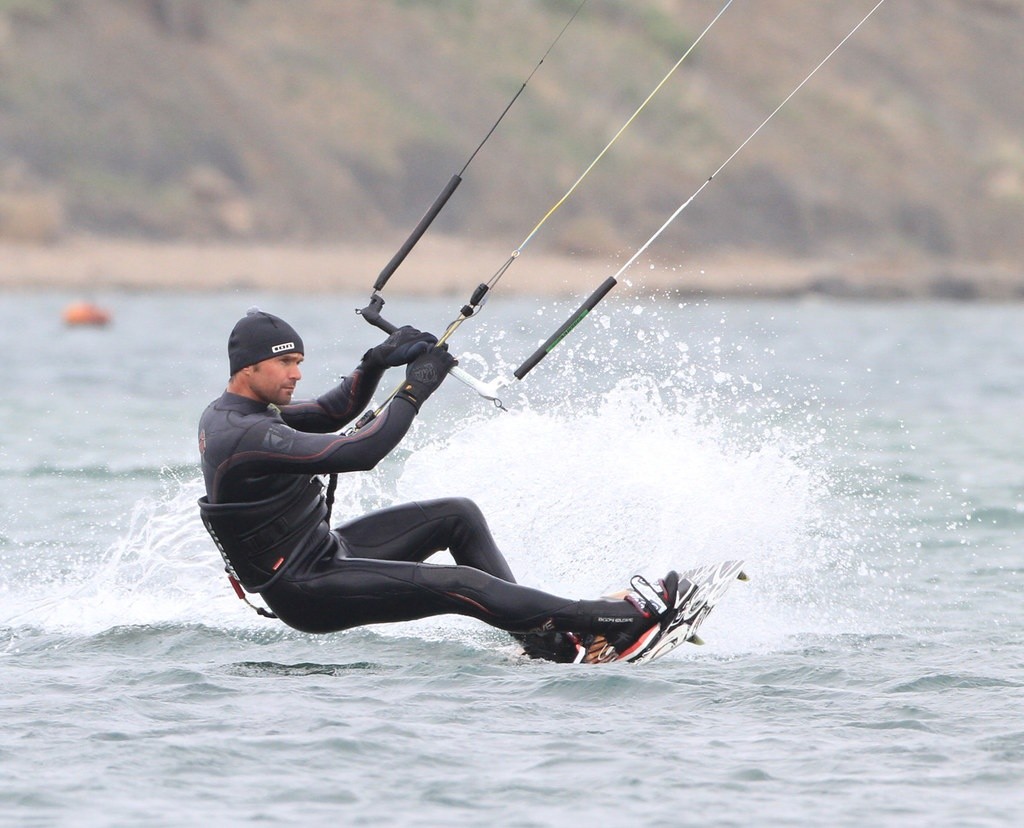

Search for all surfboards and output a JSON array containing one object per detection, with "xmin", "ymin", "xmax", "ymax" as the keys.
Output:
[{"xmin": 580, "ymin": 558, "xmax": 751, "ymax": 666}]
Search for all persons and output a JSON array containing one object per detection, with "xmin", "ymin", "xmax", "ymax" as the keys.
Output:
[{"xmin": 198, "ymin": 306, "xmax": 679, "ymax": 665}]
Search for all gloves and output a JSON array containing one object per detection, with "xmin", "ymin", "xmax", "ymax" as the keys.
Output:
[
  {"xmin": 394, "ymin": 344, "xmax": 459, "ymax": 414},
  {"xmin": 363, "ymin": 325, "xmax": 439, "ymax": 368}
]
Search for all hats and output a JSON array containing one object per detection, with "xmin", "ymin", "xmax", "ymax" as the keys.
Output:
[{"xmin": 228, "ymin": 311, "xmax": 305, "ymax": 375}]
